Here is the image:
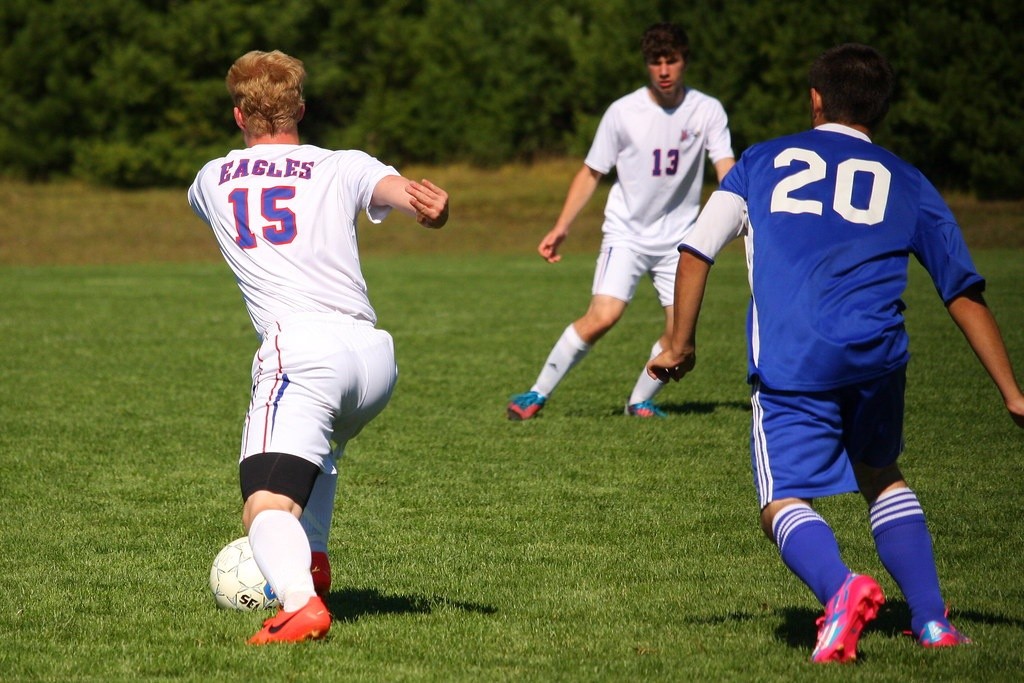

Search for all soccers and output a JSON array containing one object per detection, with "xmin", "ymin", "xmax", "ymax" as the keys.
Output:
[{"xmin": 210, "ymin": 535, "xmax": 281, "ymax": 610}]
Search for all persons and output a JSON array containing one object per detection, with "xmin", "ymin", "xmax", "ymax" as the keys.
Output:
[
  {"xmin": 185, "ymin": 50, "xmax": 450, "ymax": 646},
  {"xmin": 508, "ymin": 21, "xmax": 738, "ymax": 422},
  {"xmin": 646, "ymin": 44, "xmax": 1024, "ymax": 662}
]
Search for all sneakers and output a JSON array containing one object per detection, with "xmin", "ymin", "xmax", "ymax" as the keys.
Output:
[
  {"xmin": 246, "ymin": 596, "xmax": 331, "ymax": 644},
  {"xmin": 809, "ymin": 573, "xmax": 886, "ymax": 664},
  {"xmin": 309, "ymin": 551, "xmax": 332, "ymax": 592},
  {"xmin": 916, "ymin": 620, "xmax": 974, "ymax": 648},
  {"xmin": 624, "ymin": 399, "xmax": 668, "ymax": 419},
  {"xmin": 507, "ymin": 391, "xmax": 548, "ymax": 420}
]
